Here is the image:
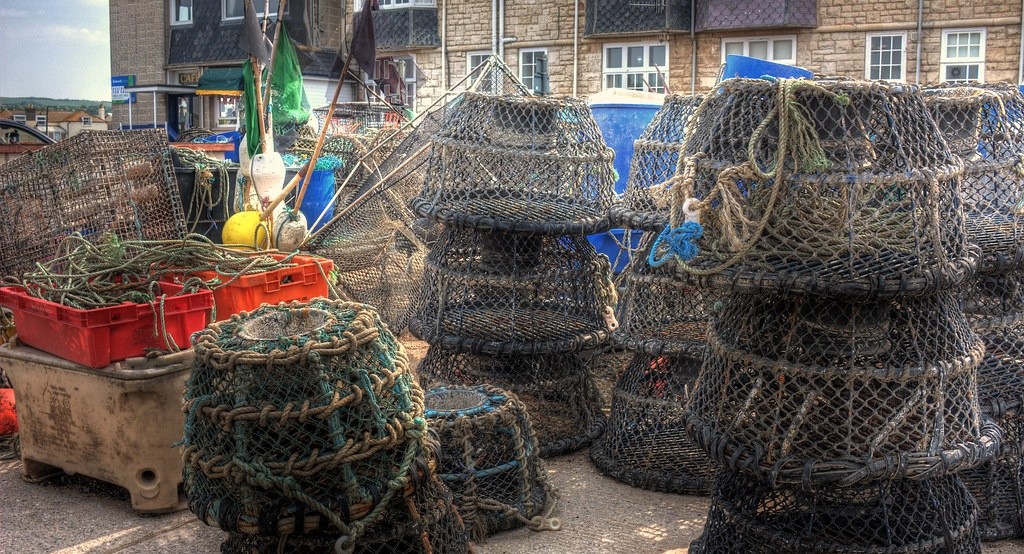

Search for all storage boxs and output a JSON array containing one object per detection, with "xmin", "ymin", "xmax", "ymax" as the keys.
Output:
[
  {"xmin": 130, "ymin": 158, "xmax": 301, "ymax": 245},
  {"xmin": 0, "ymin": 249, "xmax": 334, "ymax": 370}
]
